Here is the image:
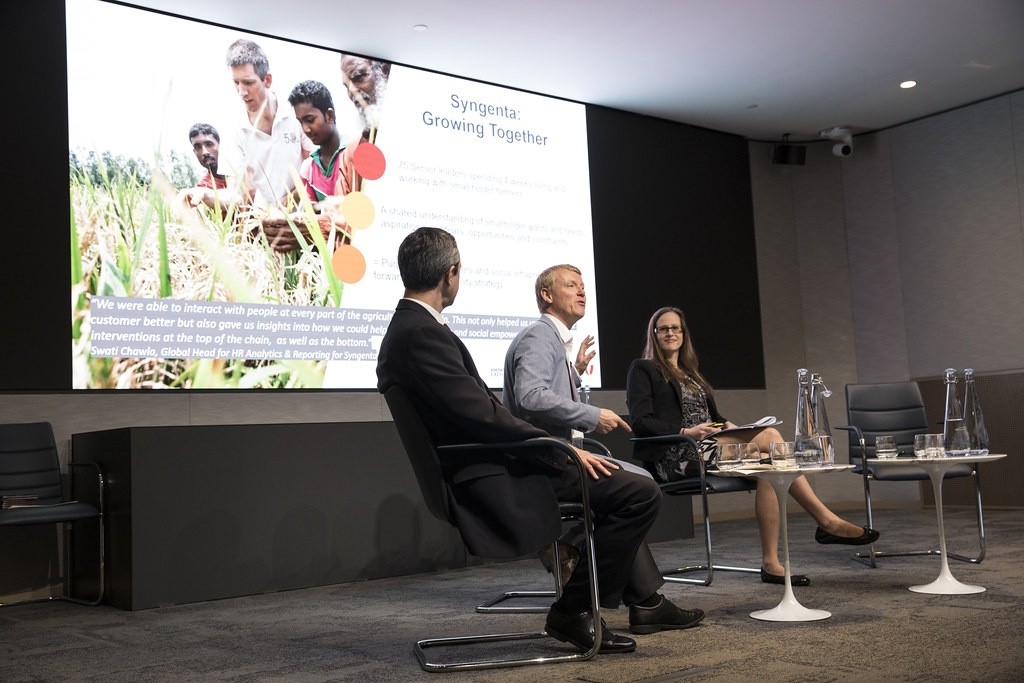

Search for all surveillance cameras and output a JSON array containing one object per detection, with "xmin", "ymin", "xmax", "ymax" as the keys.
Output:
[{"xmin": 832, "ymin": 144, "xmax": 852, "ymax": 157}]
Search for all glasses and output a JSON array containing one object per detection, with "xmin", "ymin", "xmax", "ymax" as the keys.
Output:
[{"xmin": 654, "ymin": 325, "xmax": 682, "ymax": 333}]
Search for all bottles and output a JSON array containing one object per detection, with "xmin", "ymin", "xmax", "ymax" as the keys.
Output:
[
  {"xmin": 795, "ymin": 368, "xmax": 823, "ymax": 466},
  {"xmin": 944, "ymin": 369, "xmax": 970, "ymax": 457},
  {"xmin": 810, "ymin": 372, "xmax": 835, "ymax": 465},
  {"xmin": 961, "ymin": 369, "xmax": 990, "ymax": 456}
]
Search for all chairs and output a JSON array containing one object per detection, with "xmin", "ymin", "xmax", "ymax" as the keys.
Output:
[
  {"xmin": 474, "ymin": 398, "xmax": 763, "ymax": 614},
  {"xmin": 833, "ymin": 381, "xmax": 988, "ymax": 567},
  {"xmin": 375, "ymin": 373, "xmax": 615, "ymax": 674},
  {"xmin": 0, "ymin": 421, "xmax": 106, "ymax": 606}
]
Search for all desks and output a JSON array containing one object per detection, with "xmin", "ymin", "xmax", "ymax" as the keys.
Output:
[
  {"xmin": 867, "ymin": 454, "xmax": 1008, "ymax": 595},
  {"xmin": 703, "ymin": 463, "xmax": 859, "ymax": 621}
]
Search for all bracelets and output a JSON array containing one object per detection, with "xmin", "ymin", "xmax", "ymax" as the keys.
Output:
[{"xmin": 682, "ymin": 428, "xmax": 685, "ymax": 435}]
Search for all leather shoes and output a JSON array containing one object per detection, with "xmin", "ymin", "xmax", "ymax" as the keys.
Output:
[
  {"xmin": 815, "ymin": 525, "xmax": 880, "ymax": 546},
  {"xmin": 544, "ymin": 602, "xmax": 637, "ymax": 654},
  {"xmin": 760, "ymin": 566, "xmax": 811, "ymax": 586},
  {"xmin": 629, "ymin": 594, "xmax": 705, "ymax": 635}
]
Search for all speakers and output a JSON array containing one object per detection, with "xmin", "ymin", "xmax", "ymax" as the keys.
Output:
[{"xmin": 774, "ymin": 145, "xmax": 807, "ymax": 166}]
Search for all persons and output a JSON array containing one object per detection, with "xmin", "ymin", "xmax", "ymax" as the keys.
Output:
[
  {"xmin": 176, "ymin": 38, "xmax": 392, "ymax": 264},
  {"xmin": 626, "ymin": 306, "xmax": 881, "ymax": 587},
  {"xmin": 375, "ymin": 226, "xmax": 706, "ymax": 655},
  {"xmin": 502, "ymin": 264, "xmax": 655, "ymax": 590}
]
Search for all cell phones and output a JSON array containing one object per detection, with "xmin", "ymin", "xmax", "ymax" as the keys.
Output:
[{"xmin": 711, "ymin": 423, "xmax": 724, "ymax": 428}]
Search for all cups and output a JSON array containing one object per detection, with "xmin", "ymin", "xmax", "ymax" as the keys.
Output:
[
  {"xmin": 875, "ymin": 436, "xmax": 898, "ymax": 458},
  {"xmin": 738, "ymin": 443, "xmax": 761, "ymax": 466},
  {"xmin": 771, "ymin": 441, "xmax": 795, "ymax": 467},
  {"xmin": 924, "ymin": 434, "xmax": 944, "ymax": 458},
  {"xmin": 715, "ymin": 444, "xmax": 740, "ymax": 469},
  {"xmin": 913, "ymin": 434, "xmax": 925, "ymax": 458}
]
